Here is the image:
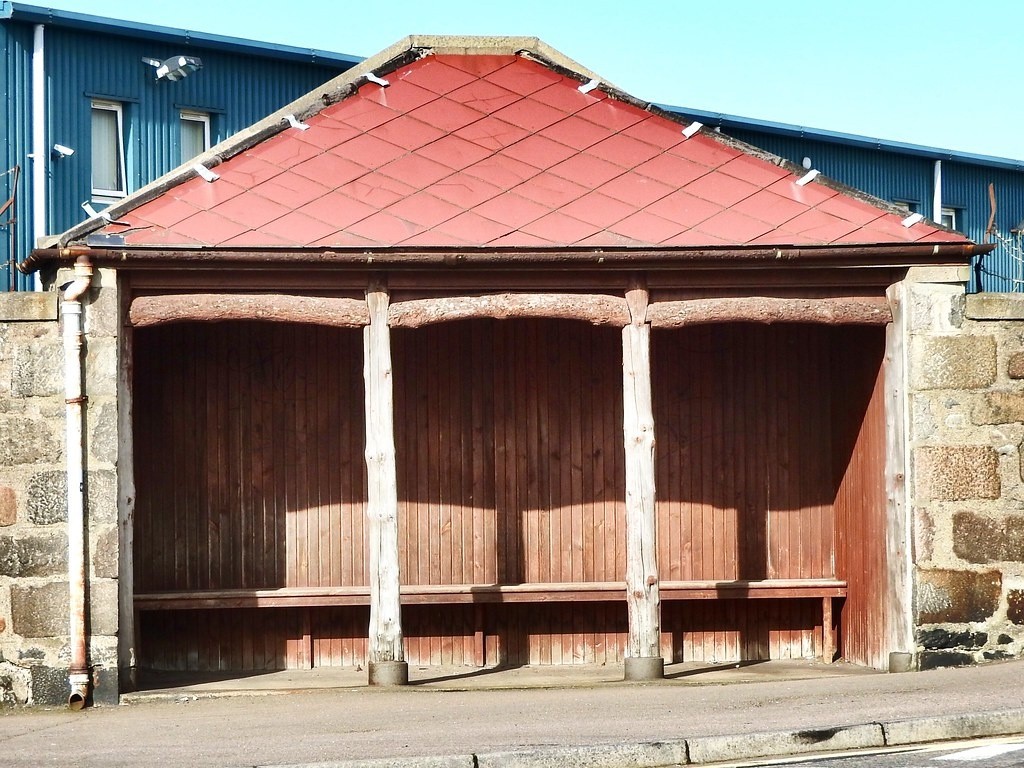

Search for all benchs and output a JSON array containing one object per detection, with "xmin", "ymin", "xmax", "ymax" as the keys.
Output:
[{"xmin": 133, "ymin": 578, "xmax": 850, "ymax": 666}]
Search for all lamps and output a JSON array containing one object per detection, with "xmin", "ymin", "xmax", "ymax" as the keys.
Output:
[{"xmin": 140, "ymin": 55, "xmax": 204, "ymax": 82}]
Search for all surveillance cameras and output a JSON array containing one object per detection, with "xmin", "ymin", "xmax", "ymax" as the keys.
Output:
[{"xmin": 53, "ymin": 144, "xmax": 75, "ymax": 157}]
